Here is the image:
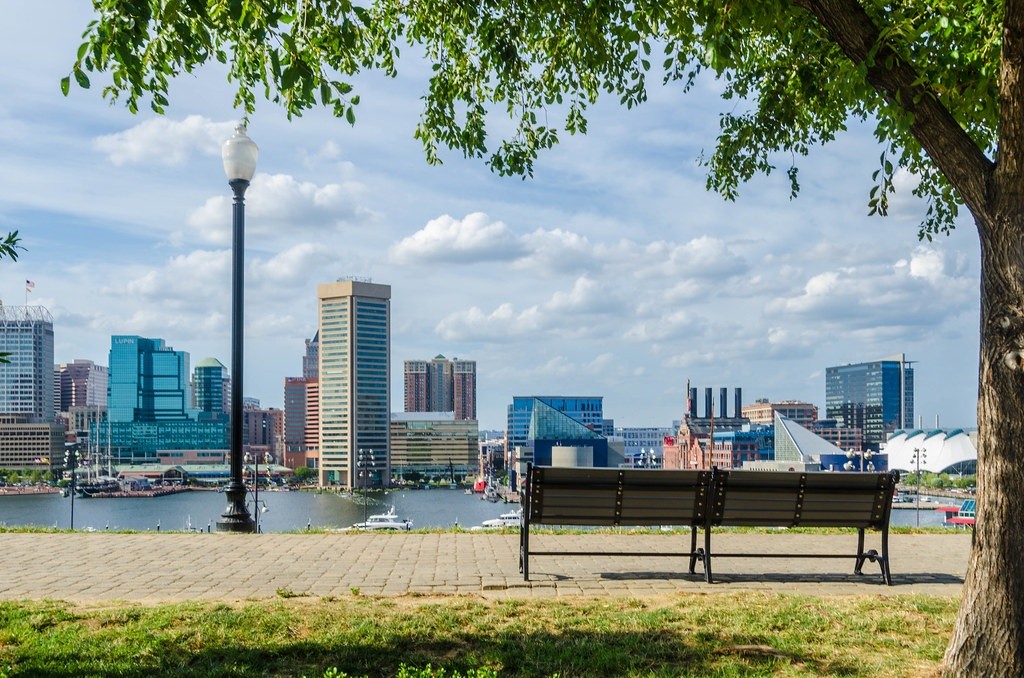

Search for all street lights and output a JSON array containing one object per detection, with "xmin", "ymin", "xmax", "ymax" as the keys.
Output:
[
  {"xmin": 244, "ymin": 451, "xmax": 275, "ymax": 533},
  {"xmin": 358, "ymin": 447, "xmax": 376, "ymax": 529},
  {"xmin": 384, "ymin": 491, "xmax": 406, "ymax": 516},
  {"xmin": 214, "ymin": 123, "xmax": 261, "ymax": 535},
  {"xmin": 910, "ymin": 447, "xmax": 927, "ymax": 528},
  {"xmin": 63, "ymin": 444, "xmax": 83, "ymax": 531}
]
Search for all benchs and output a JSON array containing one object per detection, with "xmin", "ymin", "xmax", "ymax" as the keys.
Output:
[{"xmin": 519, "ymin": 461, "xmax": 900, "ymax": 586}]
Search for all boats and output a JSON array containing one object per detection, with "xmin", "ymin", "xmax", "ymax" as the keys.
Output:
[
  {"xmin": 352, "ymin": 505, "xmax": 414, "ymax": 531},
  {"xmin": 481, "ymin": 509, "xmax": 520, "ymax": 527}
]
